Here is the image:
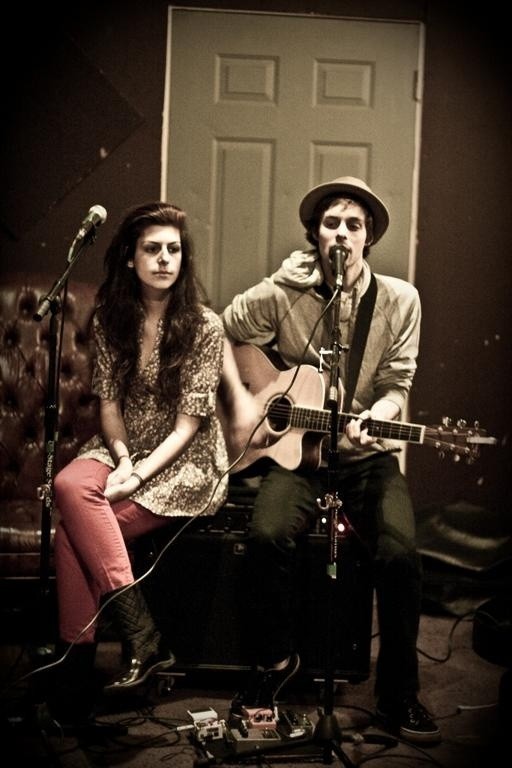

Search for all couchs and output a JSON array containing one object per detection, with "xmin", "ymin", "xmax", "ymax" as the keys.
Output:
[{"xmin": 0, "ymin": 275, "xmax": 210, "ymax": 581}]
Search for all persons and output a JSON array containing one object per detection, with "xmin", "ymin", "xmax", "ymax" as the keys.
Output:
[
  {"xmin": 218, "ymin": 175, "xmax": 443, "ymax": 742},
  {"xmin": 49, "ymin": 201, "xmax": 232, "ymax": 692}
]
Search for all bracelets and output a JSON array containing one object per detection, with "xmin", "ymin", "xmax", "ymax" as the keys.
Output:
[
  {"xmin": 131, "ymin": 472, "xmax": 144, "ymax": 487},
  {"xmin": 116, "ymin": 454, "xmax": 131, "ymax": 465}
]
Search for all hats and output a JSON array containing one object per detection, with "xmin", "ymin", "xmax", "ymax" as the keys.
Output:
[{"xmin": 297, "ymin": 175, "xmax": 390, "ymax": 245}]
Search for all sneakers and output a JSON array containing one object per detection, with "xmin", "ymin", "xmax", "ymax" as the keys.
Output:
[
  {"xmin": 376, "ymin": 696, "xmax": 442, "ymax": 744},
  {"xmin": 230, "ymin": 653, "xmax": 304, "ymax": 712}
]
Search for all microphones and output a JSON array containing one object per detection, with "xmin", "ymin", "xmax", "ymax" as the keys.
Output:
[
  {"xmin": 328, "ymin": 242, "xmax": 348, "ymax": 289},
  {"xmin": 77, "ymin": 204, "xmax": 107, "ymax": 240}
]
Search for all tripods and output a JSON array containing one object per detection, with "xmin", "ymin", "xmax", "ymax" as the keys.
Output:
[
  {"xmin": 1, "ymin": 298, "xmax": 101, "ymax": 760},
  {"xmin": 256, "ymin": 290, "xmax": 399, "ymax": 767}
]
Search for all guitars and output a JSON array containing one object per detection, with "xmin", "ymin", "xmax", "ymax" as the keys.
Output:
[{"xmin": 213, "ymin": 339, "xmax": 496, "ymax": 477}]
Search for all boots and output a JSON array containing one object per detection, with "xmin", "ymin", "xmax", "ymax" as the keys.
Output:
[{"xmin": 103, "ymin": 580, "xmax": 177, "ymax": 690}]
